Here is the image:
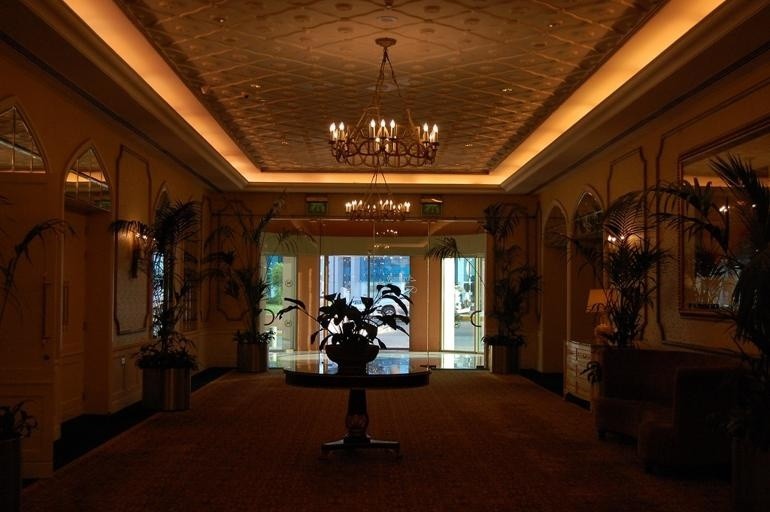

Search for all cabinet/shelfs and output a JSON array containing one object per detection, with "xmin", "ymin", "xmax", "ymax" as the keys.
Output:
[{"xmin": 563, "ymin": 341, "xmax": 594, "ymax": 412}]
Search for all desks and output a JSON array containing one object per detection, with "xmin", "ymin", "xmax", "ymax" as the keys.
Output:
[{"xmin": 282, "ymin": 357, "xmax": 433, "ymax": 461}]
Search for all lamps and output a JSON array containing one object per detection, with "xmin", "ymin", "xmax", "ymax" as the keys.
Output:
[
  {"xmin": 325, "ymin": 33, "xmax": 443, "ymax": 173},
  {"xmin": 344, "ymin": 166, "xmax": 412, "ymax": 223}
]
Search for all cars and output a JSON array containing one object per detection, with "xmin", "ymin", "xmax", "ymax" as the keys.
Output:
[{"xmin": 376, "ymin": 289, "xmax": 410, "ymax": 318}]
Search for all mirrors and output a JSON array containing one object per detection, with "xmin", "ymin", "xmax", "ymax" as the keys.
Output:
[{"xmin": 676, "ymin": 111, "xmax": 770, "ymax": 321}]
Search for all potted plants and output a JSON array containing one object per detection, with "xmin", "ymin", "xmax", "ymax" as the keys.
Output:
[
  {"xmin": 106, "ymin": 189, "xmax": 237, "ymax": 414},
  {"xmin": 274, "ymin": 284, "xmax": 416, "ymax": 367},
  {"xmin": 478, "ymin": 198, "xmax": 545, "ymax": 378},
  {"xmin": 1, "ymin": 193, "xmax": 68, "ymax": 509},
  {"xmin": 200, "ymin": 190, "xmax": 320, "ymax": 373},
  {"xmin": 648, "ymin": 138, "xmax": 770, "ymax": 510}
]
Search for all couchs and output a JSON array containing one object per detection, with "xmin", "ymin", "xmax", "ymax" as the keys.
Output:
[{"xmin": 590, "ymin": 344, "xmax": 751, "ymax": 476}]
